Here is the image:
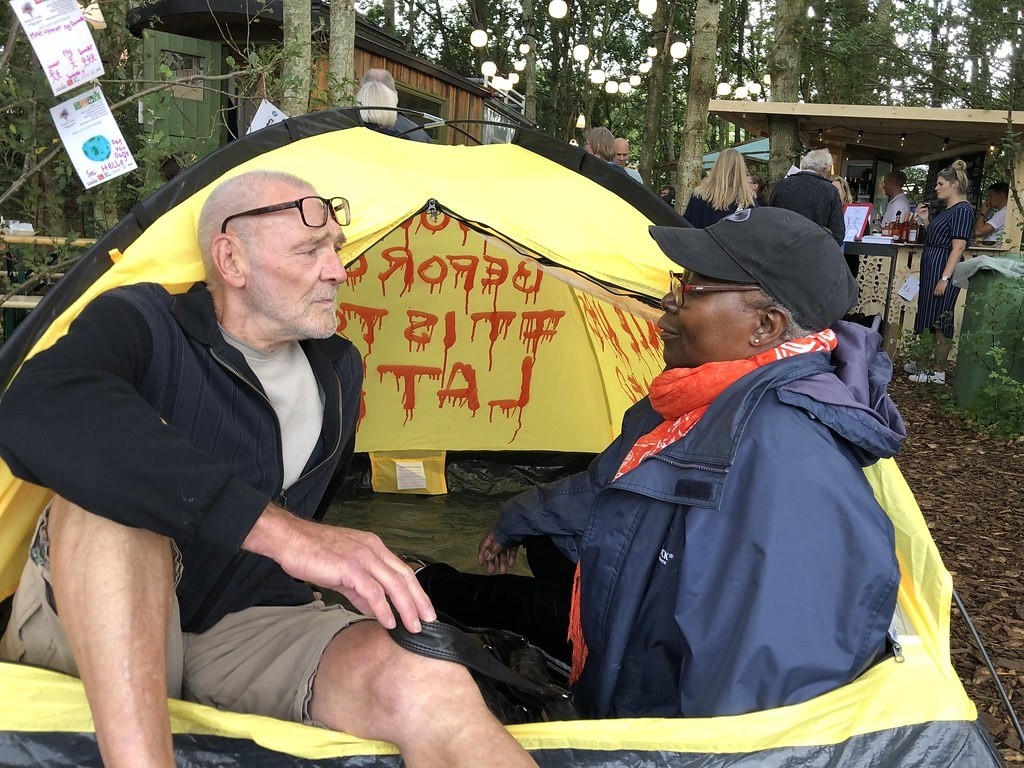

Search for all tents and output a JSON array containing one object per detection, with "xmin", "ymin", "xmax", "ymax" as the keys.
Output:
[{"xmin": 1, "ymin": 109, "xmax": 1024, "ymax": 767}]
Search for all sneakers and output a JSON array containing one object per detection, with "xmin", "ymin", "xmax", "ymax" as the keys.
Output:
[
  {"xmin": 909, "ymin": 369, "xmax": 945, "ymax": 384},
  {"xmin": 902, "ymin": 357, "xmax": 929, "ymax": 374}
]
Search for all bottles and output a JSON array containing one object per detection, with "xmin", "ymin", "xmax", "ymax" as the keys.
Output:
[
  {"xmin": 845, "ymin": 172, "xmax": 872, "ymax": 200},
  {"xmin": 874, "ymin": 209, "xmax": 926, "ymax": 245}
]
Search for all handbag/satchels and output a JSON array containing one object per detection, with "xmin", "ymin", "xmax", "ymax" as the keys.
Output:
[{"xmin": 394, "ymin": 600, "xmax": 577, "ymax": 730}]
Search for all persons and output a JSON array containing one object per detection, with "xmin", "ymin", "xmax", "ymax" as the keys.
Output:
[
  {"xmin": 747, "ymin": 174, "xmax": 767, "ymax": 206},
  {"xmin": 0, "ymin": 171, "xmax": 538, "ymax": 768},
  {"xmin": 878, "ymin": 171, "xmax": 910, "ymax": 228},
  {"xmin": 975, "ymin": 183, "xmax": 1008, "ymax": 241},
  {"xmin": 853, "ymin": 168, "xmax": 873, "ymax": 192},
  {"xmin": 660, "ymin": 186, "xmax": 675, "ymax": 199},
  {"xmin": 614, "ymin": 138, "xmax": 643, "ymax": 185},
  {"xmin": 355, "ymin": 81, "xmax": 407, "ymax": 139},
  {"xmin": 361, "ymin": 68, "xmax": 431, "ymax": 144},
  {"xmin": 682, "ymin": 148, "xmax": 759, "ymax": 229},
  {"xmin": 904, "ymin": 159, "xmax": 975, "ymax": 384},
  {"xmin": 394, "ymin": 207, "xmax": 908, "ymax": 719},
  {"xmin": 768, "ymin": 148, "xmax": 846, "ymax": 246},
  {"xmin": 584, "ymin": 125, "xmax": 627, "ymax": 173},
  {"xmin": 827, "ymin": 175, "xmax": 870, "ymax": 276}
]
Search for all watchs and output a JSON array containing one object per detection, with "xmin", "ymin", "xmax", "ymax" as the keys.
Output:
[{"xmin": 940, "ymin": 276, "xmax": 950, "ymax": 281}]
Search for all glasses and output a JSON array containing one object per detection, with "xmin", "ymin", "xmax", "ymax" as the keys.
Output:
[
  {"xmin": 217, "ymin": 188, "xmax": 352, "ymax": 239},
  {"xmin": 583, "ymin": 142, "xmax": 590, "ymax": 146},
  {"xmin": 662, "ymin": 270, "xmax": 763, "ymax": 310}
]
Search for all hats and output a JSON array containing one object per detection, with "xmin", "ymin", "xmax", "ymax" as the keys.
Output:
[{"xmin": 654, "ymin": 210, "xmax": 868, "ymax": 336}]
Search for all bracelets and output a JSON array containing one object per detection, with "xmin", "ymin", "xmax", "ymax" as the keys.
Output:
[{"xmin": 979, "ymin": 213, "xmax": 986, "ymax": 216}]
